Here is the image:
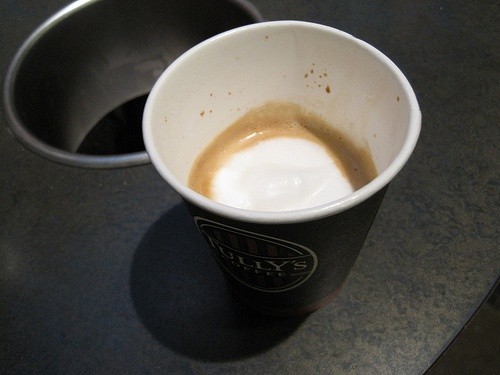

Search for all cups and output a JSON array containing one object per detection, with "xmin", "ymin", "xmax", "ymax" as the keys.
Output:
[{"xmin": 141, "ymin": 20, "xmax": 423, "ymax": 314}]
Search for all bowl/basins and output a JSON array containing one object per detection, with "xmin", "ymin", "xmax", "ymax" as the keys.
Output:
[{"xmin": 2, "ymin": 0, "xmax": 265, "ymax": 170}]
